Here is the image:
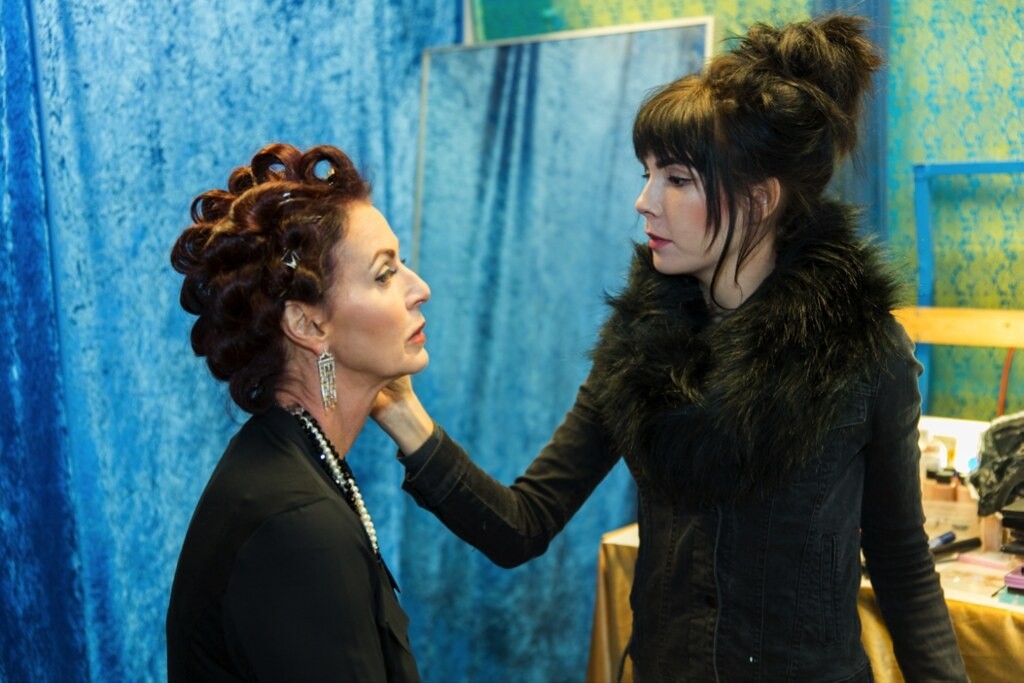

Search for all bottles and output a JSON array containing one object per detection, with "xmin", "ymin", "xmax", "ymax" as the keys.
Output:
[{"xmin": 917, "ymin": 441, "xmax": 977, "ymax": 506}]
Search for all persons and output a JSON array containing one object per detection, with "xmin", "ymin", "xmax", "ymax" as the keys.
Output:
[
  {"xmin": 372, "ymin": 12, "xmax": 970, "ymax": 683},
  {"xmin": 166, "ymin": 140, "xmax": 431, "ymax": 683}
]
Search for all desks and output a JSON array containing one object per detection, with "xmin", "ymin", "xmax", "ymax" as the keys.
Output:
[{"xmin": 584, "ymin": 523, "xmax": 1024, "ymax": 683}]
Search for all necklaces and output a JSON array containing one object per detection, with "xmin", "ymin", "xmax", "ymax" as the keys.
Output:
[{"xmin": 288, "ymin": 404, "xmax": 384, "ymax": 563}]
